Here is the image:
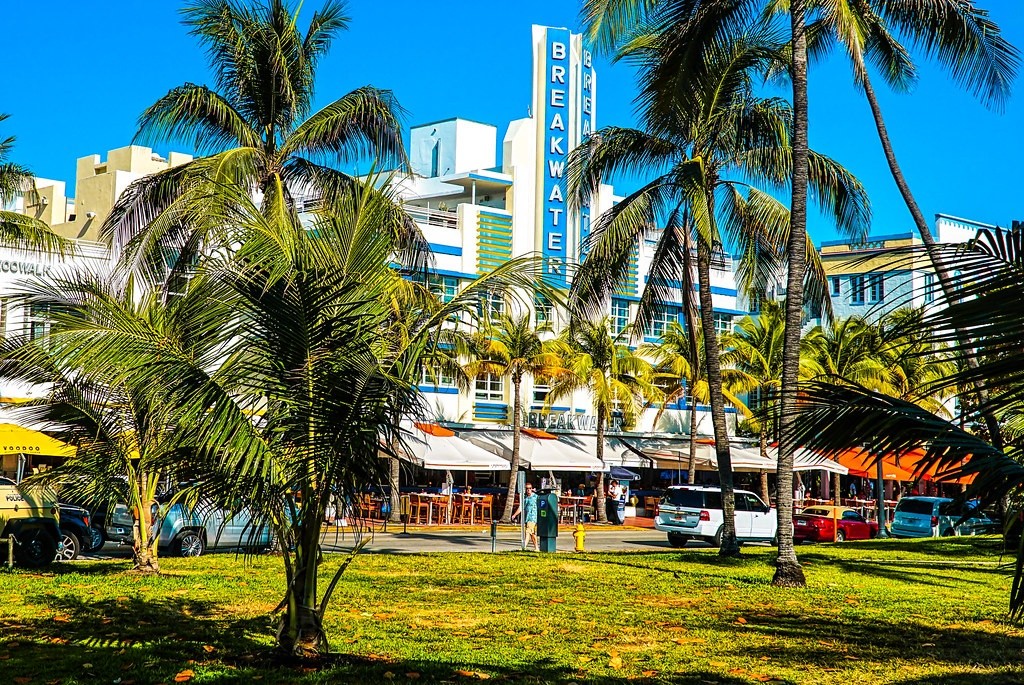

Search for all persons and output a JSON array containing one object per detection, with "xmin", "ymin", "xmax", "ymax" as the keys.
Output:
[
  {"xmin": 608, "ymin": 480, "xmax": 622, "ymax": 526},
  {"xmin": 563, "ymin": 481, "xmax": 597, "ymax": 522},
  {"xmin": 867, "ymin": 479, "xmax": 873, "ymax": 498},
  {"xmin": 512, "ymin": 483, "xmax": 539, "ymax": 551},
  {"xmin": 849, "ymin": 479, "xmax": 856, "ymax": 499}
]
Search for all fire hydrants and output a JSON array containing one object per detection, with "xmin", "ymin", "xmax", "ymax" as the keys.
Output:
[{"xmin": 572, "ymin": 523, "xmax": 588, "ymax": 553}]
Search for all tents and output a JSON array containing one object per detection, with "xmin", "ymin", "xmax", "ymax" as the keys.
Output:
[{"xmin": 0, "ymin": 423, "xmax": 190, "ymax": 491}]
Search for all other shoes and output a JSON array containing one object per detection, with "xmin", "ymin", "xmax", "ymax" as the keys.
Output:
[{"xmin": 534, "ymin": 549, "xmax": 541, "ymax": 552}]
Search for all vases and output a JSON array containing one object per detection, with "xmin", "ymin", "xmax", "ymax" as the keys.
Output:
[
  {"xmin": 807, "ymin": 497, "xmax": 810, "ymax": 499},
  {"xmin": 467, "ymin": 490, "xmax": 471, "ymax": 495}
]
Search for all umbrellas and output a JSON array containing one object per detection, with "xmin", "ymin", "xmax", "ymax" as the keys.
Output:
[{"xmin": 589, "ymin": 467, "xmax": 641, "ymax": 481}]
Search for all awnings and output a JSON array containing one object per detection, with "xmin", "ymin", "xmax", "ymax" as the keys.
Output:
[{"xmin": 376, "ymin": 423, "xmax": 979, "ymax": 522}]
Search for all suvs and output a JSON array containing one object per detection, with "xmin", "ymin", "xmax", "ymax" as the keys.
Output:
[{"xmin": 654, "ymin": 484, "xmax": 794, "ymax": 550}]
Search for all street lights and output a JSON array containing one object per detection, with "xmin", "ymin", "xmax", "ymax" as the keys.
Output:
[{"xmin": 871, "ymin": 387, "xmax": 892, "ymax": 540}]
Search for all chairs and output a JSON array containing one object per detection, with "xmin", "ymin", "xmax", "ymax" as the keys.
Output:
[
  {"xmin": 452, "ymin": 494, "xmax": 472, "ymax": 525},
  {"xmin": 364, "ymin": 495, "xmax": 380, "ymax": 519},
  {"xmin": 646, "ymin": 498, "xmax": 660, "ymax": 519},
  {"xmin": 644, "ymin": 496, "xmax": 654, "ymax": 517},
  {"xmin": 840, "ymin": 498, "xmax": 847, "ymax": 506},
  {"xmin": 847, "ymin": 501, "xmax": 859, "ymax": 507},
  {"xmin": 434, "ymin": 495, "xmax": 450, "ymax": 525},
  {"xmin": 476, "ymin": 495, "xmax": 493, "ymax": 524},
  {"xmin": 578, "ymin": 495, "xmax": 594, "ymax": 523},
  {"xmin": 355, "ymin": 494, "xmax": 368, "ymax": 518},
  {"xmin": 557, "ymin": 501, "xmax": 576, "ymax": 524},
  {"xmin": 408, "ymin": 495, "xmax": 429, "ymax": 524}
]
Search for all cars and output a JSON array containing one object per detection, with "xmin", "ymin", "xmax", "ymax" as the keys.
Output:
[
  {"xmin": 792, "ymin": 504, "xmax": 880, "ymax": 543},
  {"xmin": 105, "ymin": 480, "xmax": 304, "ymax": 558},
  {"xmin": 889, "ymin": 495, "xmax": 994, "ymax": 537},
  {"xmin": 1, "ymin": 468, "xmax": 112, "ymax": 568}
]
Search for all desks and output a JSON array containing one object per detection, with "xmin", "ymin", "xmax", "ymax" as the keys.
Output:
[
  {"xmin": 856, "ymin": 500, "xmax": 898, "ymax": 524},
  {"xmin": 421, "ymin": 495, "xmax": 445, "ymax": 525},
  {"xmin": 462, "ymin": 494, "xmax": 486, "ymax": 524},
  {"xmin": 371, "ymin": 498, "xmax": 383, "ymax": 519},
  {"xmin": 561, "ymin": 496, "xmax": 587, "ymax": 525},
  {"xmin": 807, "ymin": 499, "xmax": 835, "ymax": 507}
]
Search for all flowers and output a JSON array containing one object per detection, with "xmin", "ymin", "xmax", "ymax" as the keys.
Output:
[
  {"xmin": 467, "ymin": 485, "xmax": 472, "ymax": 491},
  {"xmin": 806, "ymin": 493, "xmax": 811, "ymax": 497}
]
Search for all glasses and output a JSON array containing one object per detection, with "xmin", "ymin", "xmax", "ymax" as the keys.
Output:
[{"xmin": 526, "ymin": 487, "xmax": 531, "ymax": 489}]
